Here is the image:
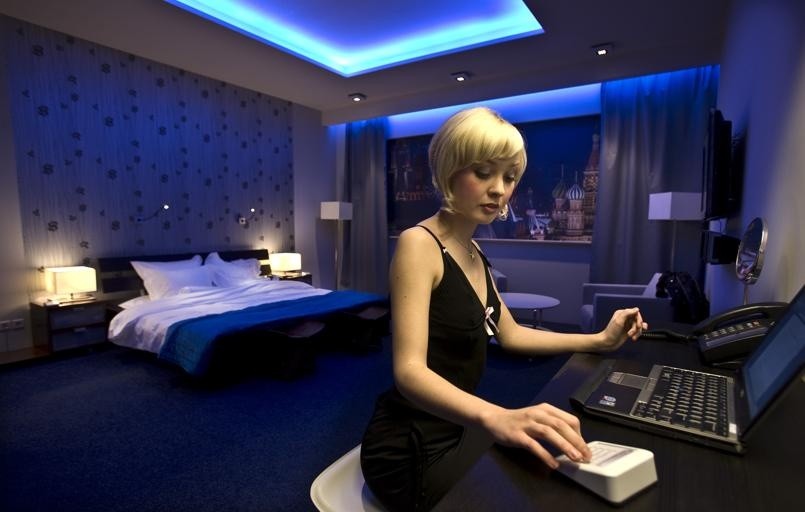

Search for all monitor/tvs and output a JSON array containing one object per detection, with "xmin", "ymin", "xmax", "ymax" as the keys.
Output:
[{"xmin": 702, "ymin": 109, "xmax": 747, "ymax": 223}]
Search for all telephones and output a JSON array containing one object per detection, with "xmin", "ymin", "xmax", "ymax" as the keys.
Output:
[{"xmin": 692, "ymin": 301, "xmax": 793, "ymax": 367}]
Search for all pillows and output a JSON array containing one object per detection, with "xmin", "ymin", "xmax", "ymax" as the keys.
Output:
[{"xmin": 130, "ymin": 252, "xmax": 261, "ymax": 302}]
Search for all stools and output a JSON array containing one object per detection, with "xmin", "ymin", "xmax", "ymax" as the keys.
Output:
[{"xmin": 309, "ymin": 443, "xmax": 388, "ymax": 512}]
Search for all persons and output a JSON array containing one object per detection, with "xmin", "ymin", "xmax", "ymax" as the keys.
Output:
[{"xmin": 359, "ymin": 106, "xmax": 648, "ymax": 512}]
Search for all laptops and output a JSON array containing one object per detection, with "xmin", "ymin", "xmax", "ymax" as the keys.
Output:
[{"xmin": 569, "ymin": 285, "xmax": 805, "ymax": 453}]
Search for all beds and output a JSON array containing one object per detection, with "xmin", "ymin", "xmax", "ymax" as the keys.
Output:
[{"xmin": 98, "ymin": 250, "xmax": 390, "ymax": 388}]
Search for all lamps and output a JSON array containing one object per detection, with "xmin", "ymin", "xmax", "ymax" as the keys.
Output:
[
  {"xmin": 270, "ymin": 253, "xmax": 302, "ymax": 278},
  {"xmin": 648, "ymin": 192, "xmax": 706, "ymax": 273},
  {"xmin": 44, "ymin": 266, "xmax": 97, "ymax": 304},
  {"xmin": 321, "ymin": 200, "xmax": 353, "ymax": 292}
]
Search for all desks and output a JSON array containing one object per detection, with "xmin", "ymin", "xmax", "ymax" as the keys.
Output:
[
  {"xmin": 416, "ymin": 323, "xmax": 805, "ymax": 512},
  {"xmin": 498, "ymin": 292, "xmax": 560, "ymax": 330}
]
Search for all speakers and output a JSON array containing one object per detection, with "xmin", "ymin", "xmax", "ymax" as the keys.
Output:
[{"xmin": 700, "ymin": 230, "xmax": 743, "ymax": 266}]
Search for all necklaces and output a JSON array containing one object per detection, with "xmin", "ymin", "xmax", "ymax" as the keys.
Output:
[{"xmin": 445, "ymin": 223, "xmax": 476, "ymax": 262}]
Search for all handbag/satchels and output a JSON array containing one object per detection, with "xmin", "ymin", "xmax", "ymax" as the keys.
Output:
[{"xmin": 655, "ymin": 269, "xmax": 710, "ymax": 324}]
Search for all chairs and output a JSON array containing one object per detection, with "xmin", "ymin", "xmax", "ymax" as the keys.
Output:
[{"xmin": 576, "ymin": 273, "xmax": 673, "ymax": 334}]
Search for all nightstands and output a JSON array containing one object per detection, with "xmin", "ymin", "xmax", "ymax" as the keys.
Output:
[
  {"xmin": 279, "ymin": 272, "xmax": 312, "ymax": 285},
  {"xmin": 30, "ymin": 298, "xmax": 109, "ymax": 352}
]
